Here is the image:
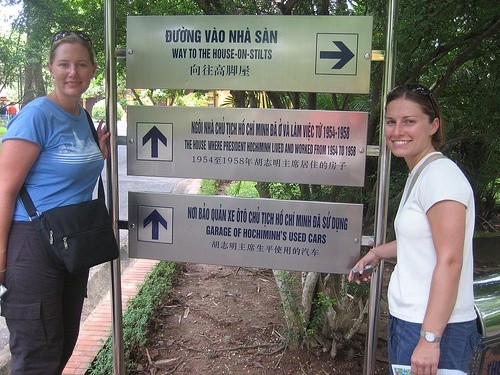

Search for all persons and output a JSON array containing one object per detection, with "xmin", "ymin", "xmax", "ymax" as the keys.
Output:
[
  {"xmin": 0, "ymin": 29, "xmax": 110, "ymax": 375},
  {"xmin": 0, "ymin": 102, "xmax": 16, "ymax": 120},
  {"xmin": 349, "ymin": 82, "xmax": 479, "ymax": 375}
]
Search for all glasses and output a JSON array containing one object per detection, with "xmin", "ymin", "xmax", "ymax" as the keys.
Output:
[
  {"xmin": 386, "ymin": 83, "xmax": 437, "ymax": 117},
  {"xmin": 52, "ymin": 30, "xmax": 92, "ymax": 51}
]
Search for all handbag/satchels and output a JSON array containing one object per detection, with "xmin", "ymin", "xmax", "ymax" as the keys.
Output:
[{"xmin": 33, "ymin": 197, "xmax": 121, "ymax": 273}]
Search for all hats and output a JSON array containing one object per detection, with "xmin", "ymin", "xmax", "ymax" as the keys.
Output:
[{"xmin": 9, "ymin": 102, "xmax": 15, "ymax": 105}]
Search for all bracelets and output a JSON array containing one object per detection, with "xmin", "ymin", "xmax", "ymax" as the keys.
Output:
[
  {"xmin": 0, "ymin": 268, "xmax": 6, "ymax": 273},
  {"xmin": 370, "ymin": 248, "xmax": 382, "ymax": 260}
]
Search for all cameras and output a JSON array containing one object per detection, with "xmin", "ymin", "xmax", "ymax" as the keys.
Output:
[{"xmin": 352, "ymin": 265, "xmax": 373, "ymax": 280}]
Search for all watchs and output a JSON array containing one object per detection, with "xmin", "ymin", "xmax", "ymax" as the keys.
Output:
[{"xmin": 420, "ymin": 329, "xmax": 442, "ymax": 343}]
